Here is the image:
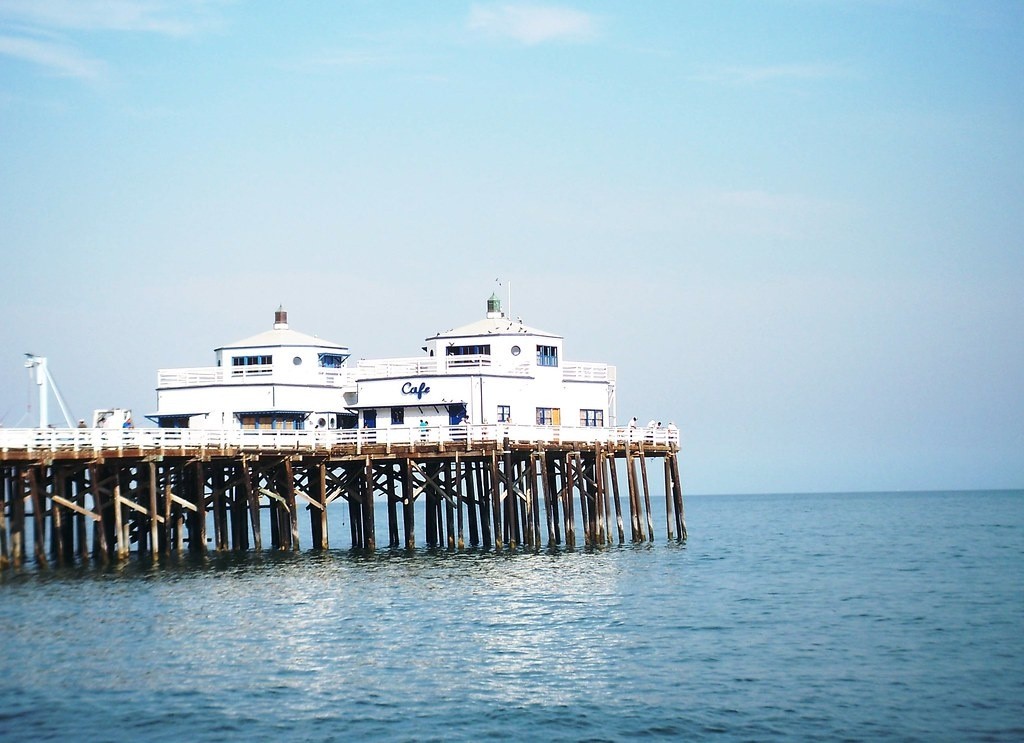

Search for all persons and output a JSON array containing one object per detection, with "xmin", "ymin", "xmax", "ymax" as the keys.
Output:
[
  {"xmin": 123, "ymin": 417, "xmax": 135, "ymax": 447},
  {"xmin": 646, "ymin": 420, "xmax": 662, "ymax": 443},
  {"xmin": 628, "ymin": 416, "xmax": 638, "ymax": 444},
  {"xmin": 418, "ymin": 419, "xmax": 430, "ymax": 444},
  {"xmin": 98, "ymin": 417, "xmax": 105, "ymax": 444},
  {"xmin": 459, "ymin": 415, "xmax": 473, "ymax": 441},
  {"xmin": 76, "ymin": 419, "xmax": 88, "ymax": 441}
]
